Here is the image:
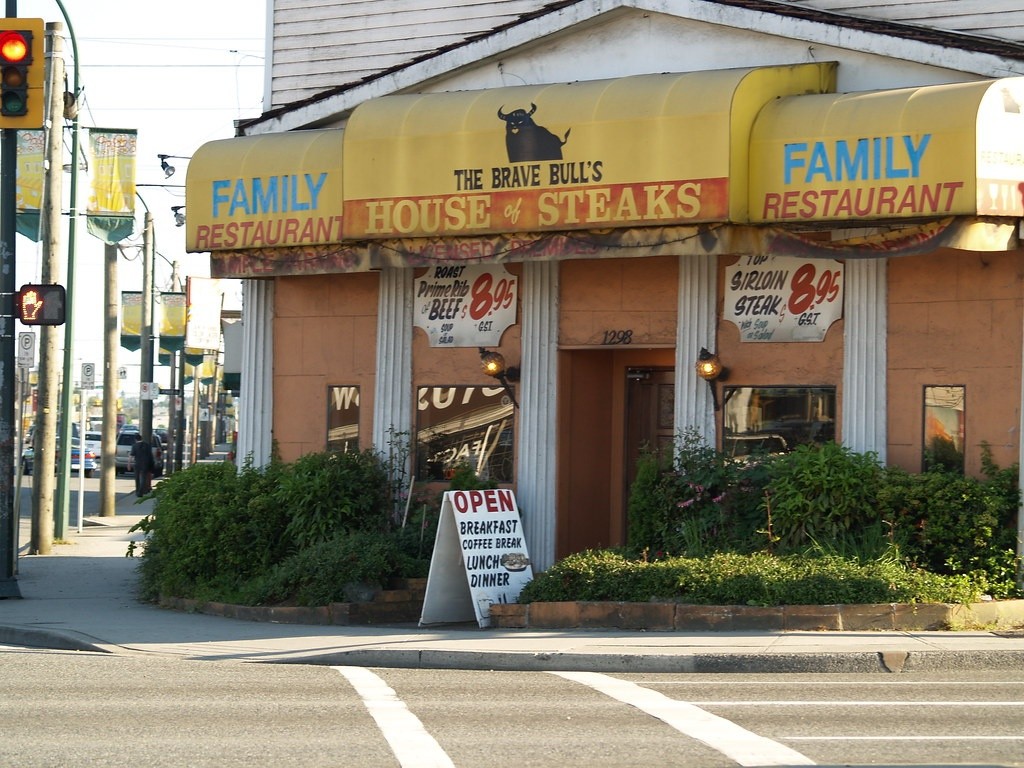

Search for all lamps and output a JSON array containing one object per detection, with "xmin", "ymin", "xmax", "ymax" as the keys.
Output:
[
  {"xmin": 158, "ymin": 154, "xmax": 192, "ymax": 180},
  {"xmin": 171, "ymin": 206, "xmax": 186, "ymax": 227},
  {"xmin": 477, "ymin": 345, "xmax": 522, "ymax": 411},
  {"xmin": 695, "ymin": 346, "xmax": 730, "ymax": 410}
]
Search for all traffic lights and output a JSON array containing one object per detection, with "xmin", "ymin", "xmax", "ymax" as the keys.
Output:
[
  {"xmin": 0, "ymin": 17, "xmax": 45, "ymax": 131},
  {"xmin": 16, "ymin": 283, "xmax": 67, "ymax": 328}
]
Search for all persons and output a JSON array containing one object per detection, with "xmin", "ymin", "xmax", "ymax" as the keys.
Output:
[{"xmin": 130, "ymin": 434, "xmax": 151, "ymax": 497}]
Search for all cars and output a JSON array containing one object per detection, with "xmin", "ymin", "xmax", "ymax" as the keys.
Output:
[
  {"xmin": 22, "ymin": 436, "xmax": 98, "ymax": 477},
  {"xmin": 86, "ymin": 430, "xmax": 102, "ymax": 457},
  {"xmin": 154, "ymin": 427, "xmax": 167, "ymax": 443},
  {"xmin": 117, "ymin": 422, "xmax": 139, "ymax": 432},
  {"xmin": 116, "ymin": 429, "xmax": 167, "ymax": 477}
]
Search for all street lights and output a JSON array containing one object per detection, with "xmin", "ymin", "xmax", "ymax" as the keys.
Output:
[
  {"xmin": 116, "ymin": 241, "xmax": 188, "ymax": 471},
  {"xmin": 63, "ymin": 162, "xmax": 155, "ymax": 488}
]
